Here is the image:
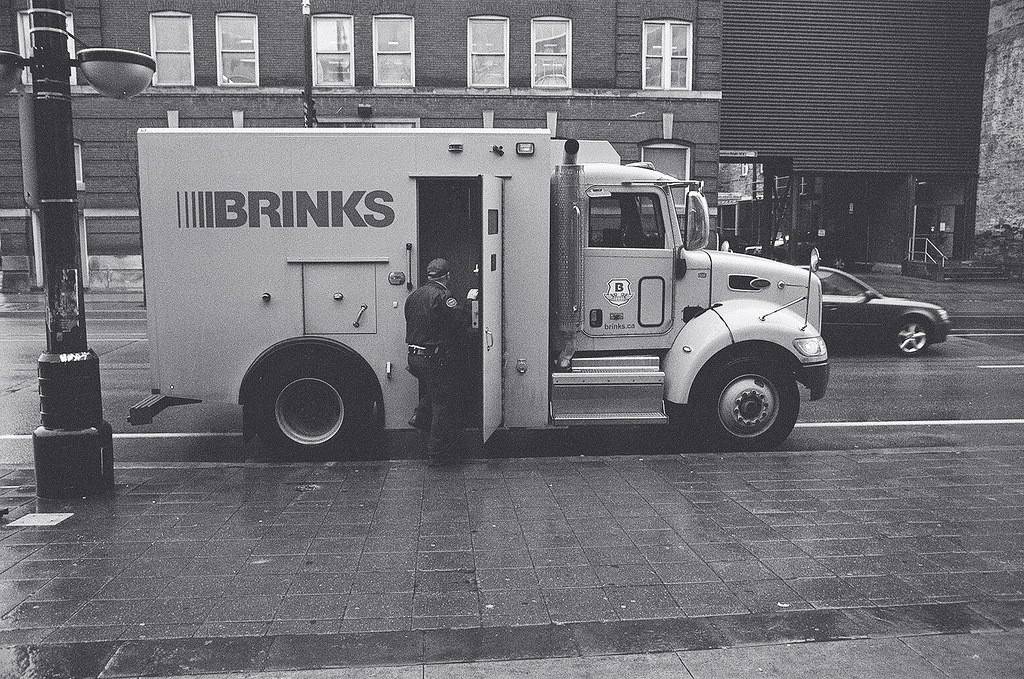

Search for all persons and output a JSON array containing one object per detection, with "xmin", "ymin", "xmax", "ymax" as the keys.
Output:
[{"xmin": 405, "ymin": 258, "xmax": 480, "ymax": 465}]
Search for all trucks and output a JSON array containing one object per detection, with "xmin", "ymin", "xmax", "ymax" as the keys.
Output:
[{"xmin": 126, "ymin": 126, "xmax": 830, "ymax": 461}]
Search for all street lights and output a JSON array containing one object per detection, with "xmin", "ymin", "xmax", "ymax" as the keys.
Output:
[{"xmin": 0, "ymin": 0, "xmax": 159, "ymax": 497}]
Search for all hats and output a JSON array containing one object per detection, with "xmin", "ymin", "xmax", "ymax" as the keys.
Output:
[{"xmin": 426, "ymin": 257, "xmax": 450, "ymax": 276}]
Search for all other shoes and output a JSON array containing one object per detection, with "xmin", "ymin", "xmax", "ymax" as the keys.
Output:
[{"xmin": 409, "ymin": 414, "xmax": 427, "ymax": 432}]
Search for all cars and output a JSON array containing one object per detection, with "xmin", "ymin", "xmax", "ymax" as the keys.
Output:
[{"xmin": 798, "ymin": 265, "xmax": 950, "ymax": 357}]
